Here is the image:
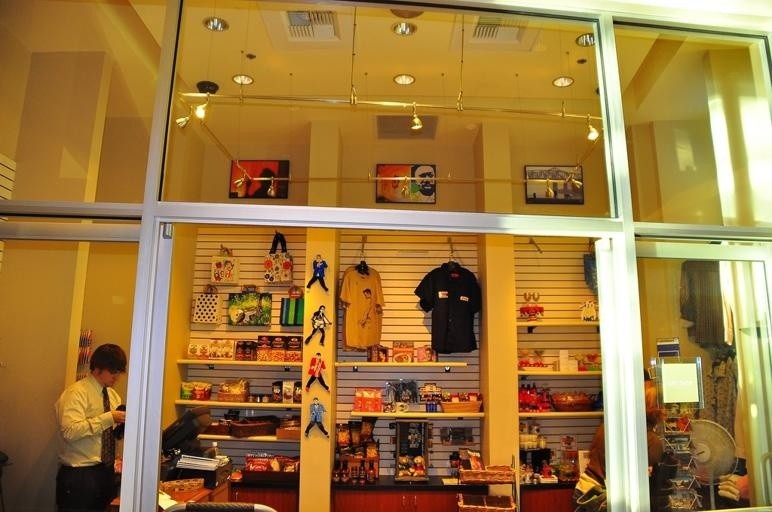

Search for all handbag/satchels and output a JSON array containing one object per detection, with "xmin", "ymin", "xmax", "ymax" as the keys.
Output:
[{"xmin": 193, "ymin": 232, "xmax": 304, "ymax": 326}]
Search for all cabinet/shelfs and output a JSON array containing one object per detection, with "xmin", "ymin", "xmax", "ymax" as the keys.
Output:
[
  {"xmin": 662, "ymin": 411, "xmax": 703, "ymax": 470},
  {"xmin": 110, "ymin": 481, "xmax": 228, "ymax": 510},
  {"xmin": 520, "ymin": 319, "xmax": 604, "ymax": 418}
]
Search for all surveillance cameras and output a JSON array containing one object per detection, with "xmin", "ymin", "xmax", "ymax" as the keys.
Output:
[{"xmin": 196, "ymin": 81, "xmax": 219, "ymax": 94}]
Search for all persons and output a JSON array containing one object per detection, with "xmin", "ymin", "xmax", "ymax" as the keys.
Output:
[
  {"xmin": 307, "ymin": 255, "xmax": 329, "ymax": 296},
  {"xmin": 305, "ymin": 305, "xmax": 329, "ymax": 346},
  {"xmin": 410, "ymin": 164, "xmax": 436, "ymax": 203},
  {"xmin": 244, "ymin": 168, "xmax": 275, "ymax": 199},
  {"xmin": 571, "ymin": 370, "xmax": 679, "ymax": 512},
  {"xmin": 53, "ymin": 344, "xmax": 127, "ymax": 512},
  {"xmin": 305, "ymin": 353, "xmax": 330, "ymax": 392},
  {"xmin": 304, "ymin": 398, "xmax": 329, "ymax": 439}
]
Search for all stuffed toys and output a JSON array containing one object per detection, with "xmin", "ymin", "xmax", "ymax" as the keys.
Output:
[{"xmin": 398, "ymin": 456, "xmax": 426, "ymax": 477}]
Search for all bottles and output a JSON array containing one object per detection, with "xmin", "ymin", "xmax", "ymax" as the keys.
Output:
[
  {"xmin": 332, "ymin": 460, "xmax": 376, "ymax": 486},
  {"xmin": 426, "ymin": 401, "xmax": 437, "ymax": 412},
  {"xmin": 246, "ymin": 392, "xmax": 271, "ymax": 402},
  {"xmin": 212, "ymin": 442, "xmax": 220, "ymax": 455}
]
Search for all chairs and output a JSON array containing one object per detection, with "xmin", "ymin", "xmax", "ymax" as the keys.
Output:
[
  {"xmin": 175, "ymin": 359, "xmax": 301, "ymax": 443},
  {"xmin": 335, "ymin": 361, "xmax": 486, "ymax": 419}
]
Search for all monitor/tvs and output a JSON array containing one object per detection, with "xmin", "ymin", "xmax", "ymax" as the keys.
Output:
[{"xmin": 161, "ymin": 406, "xmax": 217, "ymax": 471}]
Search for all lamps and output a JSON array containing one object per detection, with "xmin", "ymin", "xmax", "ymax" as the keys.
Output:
[
  {"xmin": 572, "ymin": 179, "xmax": 582, "ymax": 189},
  {"xmin": 401, "ymin": 180, "xmax": 411, "ymax": 198},
  {"xmin": 410, "ymin": 106, "xmax": 422, "ymax": 131},
  {"xmin": 545, "ymin": 184, "xmax": 554, "ymax": 197},
  {"xmin": 176, "ymin": 117, "xmax": 191, "ymax": 128},
  {"xmin": 266, "ymin": 180, "xmax": 277, "ymax": 196},
  {"xmin": 586, "ymin": 119, "xmax": 599, "ymax": 141},
  {"xmin": 194, "ymin": 96, "xmax": 210, "ymax": 119},
  {"xmin": 234, "ymin": 176, "xmax": 247, "ymax": 188}
]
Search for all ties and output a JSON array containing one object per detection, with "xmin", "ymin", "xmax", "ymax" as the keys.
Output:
[{"xmin": 101, "ymin": 386, "xmax": 116, "ymax": 470}]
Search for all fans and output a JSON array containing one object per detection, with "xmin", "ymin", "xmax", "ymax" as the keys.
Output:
[{"xmin": 690, "ymin": 420, "xmax": 737, "ymax": 510}]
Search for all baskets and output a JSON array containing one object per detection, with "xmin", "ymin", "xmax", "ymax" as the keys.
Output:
[
  {"xmin": 440, "ymin": 399, "xmax": 482, "ymax": 412},
  {"xmin": 218, "ymin": 380, "xmax": 249, "ymax": 403},
  {"xmin": 458, "ymin": 493, "xmax": 516, "ymax": 512},
  {"xmin": 552, "ymin": 401, "xmax": 596, "ymax": 412},
  {"xmin": 459, "ymin": 465, "xmax": 516, "ymax": 485}
]
